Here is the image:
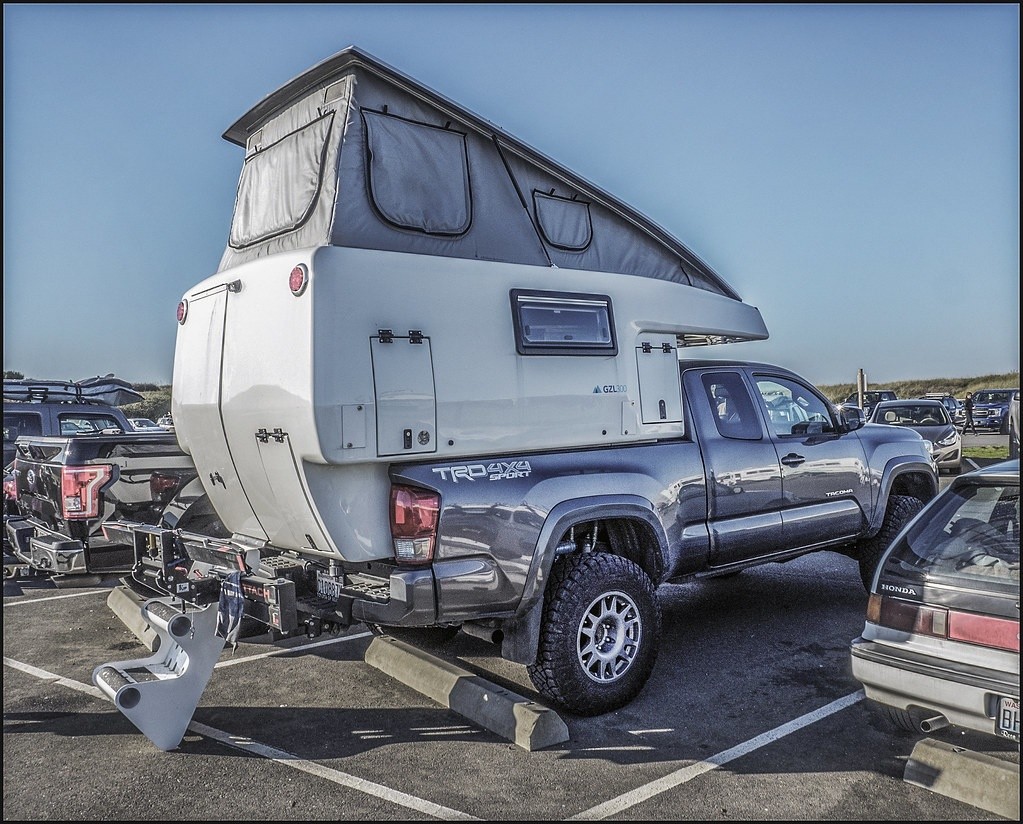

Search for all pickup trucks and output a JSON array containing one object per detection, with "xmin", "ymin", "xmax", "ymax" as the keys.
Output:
[
  {"xmin": 98, "ymin": 357, "xmax": 941, "ymax": 717},
  {"xmin": 1, "ymin": 432, "xmax": 232, "ymax": 580}
]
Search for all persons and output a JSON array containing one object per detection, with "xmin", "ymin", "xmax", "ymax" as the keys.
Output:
[
  {"xmin": 959, "ymin": 392, "xmax": 980, "ymax": 436},
  {"xmin": 866, "ymin": 394, "xmax": 877, "ymax": 416},
  {"xmin": 917, "ymin": 410, "xmax": 939, "ymax": 423}
]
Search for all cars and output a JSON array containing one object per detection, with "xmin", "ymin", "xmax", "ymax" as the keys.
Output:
[
  {"xmin": 845, "ymin": 456, "xmax": 1020, "ymax": 748},
  {"xmin": 61, "ymin": 420, "xmax": 87, "ymax": 437},
  {"xmin": 765, "ymin": 402, "xmax": 810, "ymax": 423},
  {"xmin": 76, "ymin": 415, "xmax": 175, "ymax": 434},
  {"xmin": 835, "ymin": 386, "xmax": 1019, "ymax": 435},
  {"xmin": 1008, "ymin": 389, "xmax": 1021, "ymax": 458},
  {"xmin": 868, "ymin": 399, "xmax": 965, "ymax": 474}
]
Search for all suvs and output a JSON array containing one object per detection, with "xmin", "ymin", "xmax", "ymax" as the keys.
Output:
[{"xmin": 1, "ymin": 393, "xmax": 135, "ymax": 468}]
